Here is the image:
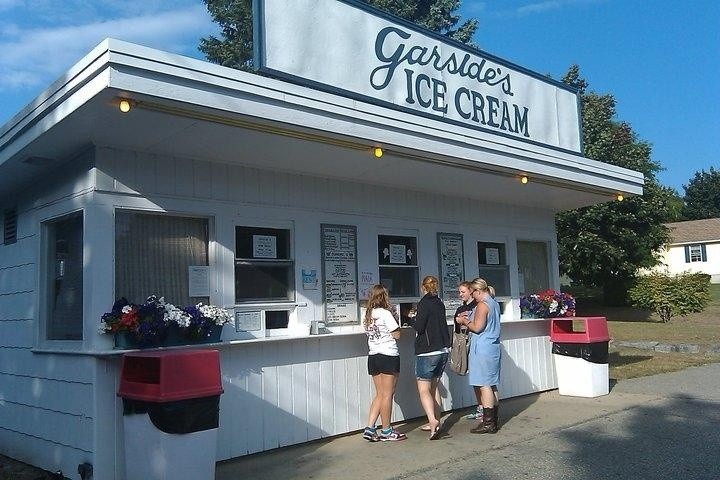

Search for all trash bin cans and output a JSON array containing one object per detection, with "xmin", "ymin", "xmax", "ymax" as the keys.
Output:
[
  {"xmin": 549, "ymin": 317, "xmax": 611, "ymax": 398},
  {"xmin": 116, "ymin": 350, "xmax": 225, "ymax": 480}
]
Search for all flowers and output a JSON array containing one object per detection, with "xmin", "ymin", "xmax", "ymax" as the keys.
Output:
[
  {"xmin": 519, "ymin": 288, "xmax": 576, "ymax": 318},
  {"xmin": 98, "ymin": 295, "xmax": 190, "ymax": 345},
  {"xmin": 177, "ymin": 303, "xmax": 233, "ymax": 340}
]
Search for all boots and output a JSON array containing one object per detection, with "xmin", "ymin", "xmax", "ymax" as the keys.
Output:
[{"xmin": 470, "ymin": 407, "xmax": 498, "ymax": 435}]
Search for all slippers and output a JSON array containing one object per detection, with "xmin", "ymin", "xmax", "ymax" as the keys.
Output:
[
  {"xmin": 420, "ymin": 424, "xmax": 430, "ymax": 431},
  {"xmin": 430, "ymin": 424, "xmax": 444, "ymax": 440}
]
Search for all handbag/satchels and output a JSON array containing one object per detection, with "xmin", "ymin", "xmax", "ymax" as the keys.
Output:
[{"xmin": 449, "ymin": 331, "xmax": 470, "ymax": 376}]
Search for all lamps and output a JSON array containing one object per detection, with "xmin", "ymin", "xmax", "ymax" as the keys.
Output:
[
  {"xmin": 521, "ymin": 174, "xmax": 528, "ymax": 184},
  {"xmin": 374, "ymin": 146, "xmax": 382, "ymax": 158},
  {"xmin": 615, "ymin": 193, "xmax": 624, "ymax": 202},
  {"xmin": 120, "ymin": 99, "xmax": 131, "ymax": 113}
]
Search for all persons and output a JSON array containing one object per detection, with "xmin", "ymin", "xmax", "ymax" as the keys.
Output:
[
  {"xmin": 407, "ymin": 276, "xmax": 451, "ymax": 440},
  {"xmin": 363, "ymin": 285, "xmax": 408, "ymax": 442},
  {"xmin": 454, "ymin": 278, "xmax": 501, "ymax": 434}
]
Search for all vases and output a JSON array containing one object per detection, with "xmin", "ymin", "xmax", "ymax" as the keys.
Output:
[
  {"xmin": 165, "ymin": 322, "xmax": 223, "ymax": 346},
  {"xmin": 113, "ymin": 330, "xmax": 169, "ymax": 349}
]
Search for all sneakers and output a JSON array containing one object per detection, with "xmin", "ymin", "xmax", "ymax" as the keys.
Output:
[
  {"xmin": 362, "ymin": 426, "xmax": 406, "ymax": 442},
  {"xmin": 466, "ymin": 407, "xmax": 483, "ymax": 420}
]
